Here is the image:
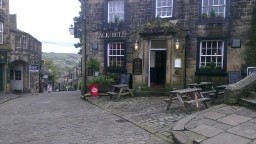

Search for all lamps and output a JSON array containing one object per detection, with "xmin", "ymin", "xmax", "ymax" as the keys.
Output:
[
  {"xmin": 175, "ymin": 39, "xmax": 180, "ymax": 50},
  {"xmin": 68, "ymin": 24, "xmax": 75, "ymax": 35},
  {"xmin": 134, "ymin": 40, "xmax": 139, "ymax": 50}
]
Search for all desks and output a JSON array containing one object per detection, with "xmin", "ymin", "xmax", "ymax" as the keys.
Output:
[
  {"xmin": 163, "ymin": 81, "xmax": 232, "ymax": 109},
  {"xmin": 107, "ymin": 84, "xmax": 136, "ymax": 101}
]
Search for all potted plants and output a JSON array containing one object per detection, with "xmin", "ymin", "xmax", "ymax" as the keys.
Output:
[
  {"xmin": 104, "ymin": 58, "xmax": 127, "ymax": 74},
  {"xmin": 194, "ymin": 58, "xmax": 229, "ymax": 76}
]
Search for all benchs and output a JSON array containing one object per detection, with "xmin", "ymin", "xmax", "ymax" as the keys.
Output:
[
  {"xmin": 108, "ymin": 92, "xmax": 117, "ymax": 96},
  {"xmin": 165, "ymin": 97, "xmax": 185, "ymax": 102},
  {"xmin": 126, "ymin": 89, "xmax": 135, "ymax": 92},
  {"xmin": 210, "ymin": 92, "xmax": 225, "ymax": 97},
  {"xmin": 200, "ymin": 91, "xmax": 217, "ymax": 95},
  {"xmin": 185, "ymin": 98, "xmax": 211, "ymax": 105}
]
